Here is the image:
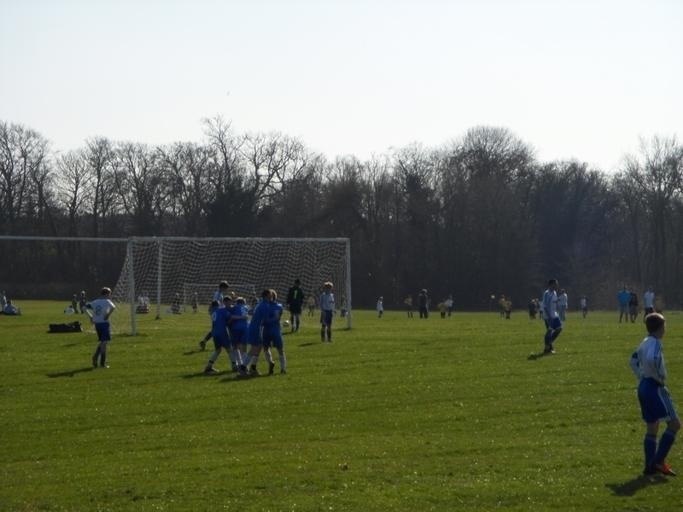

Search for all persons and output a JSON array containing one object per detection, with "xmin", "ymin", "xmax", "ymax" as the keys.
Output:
[
  {"xmin": 341, "ymin": 295, "xmax": 348, "ymax": 318},
  {"xmin": 239, "ymin": 289, "xmax": 281, "ymax": 374},
  {"xmin": 192, "ymin": 292, "xmax": 199, "ymax": 313},
  {"xmin": 138, "ymin": 290, "xmax": 146, "ymax": 314},
  {"xmin": 419, "ymin": 288, "xmax": 430, "ymax": 319},
  {"xmin": 266, "ymin": 289, "xmax": 287, "ymax": 375},
  {"xmin": 423, "ymin": 288, "xmax": 430, "ymax": 318},
  {"xmin": 618, "ymin": 285, "xmax": 633, "ymax": 323},
  {"xmin": 81, "ymin": 290, "xmax": 91, "ymax": 314},
  {"xmin": 629, "ymin": 286, "xmax": 640, "ymax": 324},
  {"xmin": 504, "ymin": 296, "xmax": 513, "ymax": 320},
  {"xmin": 558, "ymin": 288, "xmax": 568, "ymax": 322},
  {"xmin": 249, "ymin": 291, "xmax": 260, "ymax": 316},
  {"xmin": 438, "ymin": 298, "xmax": 448, "ymax": 319},
  {"xmin": 230, "ymin": 291, "xmax": 238, "ymax": 304},
  {"xmin": 3, "ymin": 299, "xmax": 22, "ymax": 315},
  {"xmin": 581, "ymin": 295, "xmax": 589, "ymax": 319},
  {"xmin": 64, "ymin": 305, "xmax": 75, "ymax": 315},
  {"xmin": 405, "ymin": 294, "xmax": 415, "ymax": 319},
  {"xmin": 1, "ymin": 289, "xmax": 10, "ymax": 314},
  {"xmin": 223, "ymin": 296, "xmax": 243, "ymax": 371},
  {"xmin": 321, "ymin": 282, "xmax": 336, "ymax": 343},
  {"xmin": 499, "ymin": 294, "xmax": 507, "ymax": 318},
  {"xmin": 172, "ymin": 292, "xmax": 182, "ymax": 315},
  {"xmin": 73, "ymin": 294, "xmax": 80, "ymax": 313},
  {"xmin": 288, "ymin": 279, "xmax": 305, "ymax": 335},
  {"xmin": 535, "ymin": 297, "xmax": 541, "ymax": 314},
  {"xmin": 92, "ymin": 286, "xmax": 117, "ymax": 369},
  {"xmin": 308, "ymin": 292, "xmax": 316, "ymax": 317},
  {"xmin": 203, "ymin": 300, "xmax": 250, "ymax": 374},
  {"xmin": 229, "ymin": 297, "xmax": 250, "ymax": 372},
  {"xmin": 559, "ymin": 292, "xmax": 569, "ymax": 323},
  {"xmin": 644, "ymin": 285, "xmax": 657, "ymax": 324},
  {"xmin": 231, "ymin": 291, "xmax": 250, "ymax": 371},
  {"xmin": 543, "ymin": 278, "xmax": 564, "ymax": 354},
  {"xmin": 630, "ymin": 313, "xmax": 681, "ymax": 477},
  {"xmin": 446, "ymin": 294, "xmax": 453, "ymax": 317},
  {"xmin": 378, "ymin": 296, "xmax": 385, "ymax": 319},
  {"xmin": 200, "ymin": 281, "xmax": 230, "ymax": 350},
  {"xmin": 530, "ymin": 299, "xmax": 538, "ymax": 319},
  {"xmin": 144, "ymin": 291, "xmax": 150, "ymax": 313},
  {"xmin": 539, "ymin": 297, "xmax": 545, "ymax": 319}
]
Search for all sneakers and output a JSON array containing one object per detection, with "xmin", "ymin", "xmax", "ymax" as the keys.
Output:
[
  {"xmin": 232, "ymin": 365, "xmax": 237, "ymax": 371},
  {"xmin": 239, "ymin": 367, "xmax": 249, "ymax": 376},
  {"xmin": 269, "ymin": 362, "xmax": 276, "ymax": 374},
  {"xmin": 250, "ymin": 368, "xmax": 261, "ymax": 375},
  {"xmin": 200, "ymin": 341, "xmax": 205, "ymax": 350},
  {"xmin": 545, "ymin": 349, "xmax": 554, "ymax": 353},
  {"xmin": 204, "ymin": 368, "xmax": 217, "ymax": 374},
  {"xmin": 100, "ymin": 365, "xmax": 110, "ymax": 368},
  {"xmin": 281, "ymin": 369, "xmax": 285, "ymax": 373},
  {"xmin": 644, "ymin": 466, "xmax": 657, "ymax": 476},
  {"xmin": 653, "ymin": 461, "xmax": 674, "ymax": 475},
  {"xmin": 92, "ymin": 356, "xmax": 97, "ymax": 367}
]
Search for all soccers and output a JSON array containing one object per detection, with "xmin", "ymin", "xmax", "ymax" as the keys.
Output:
[{"xmin": 282, "ymin": 320, "xmax": 289, "ymax": 326}]
